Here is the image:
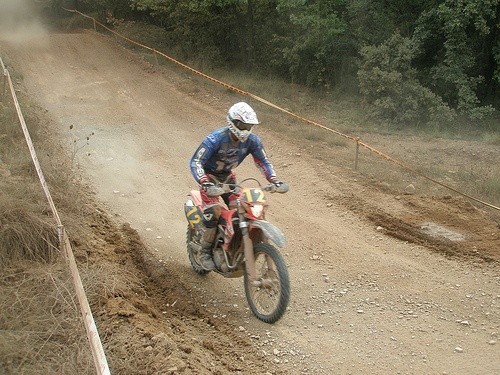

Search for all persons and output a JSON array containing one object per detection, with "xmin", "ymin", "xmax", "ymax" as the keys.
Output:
[{"xmin": 189, "ymin": 102, "xmax": 284, "ymax": 271}]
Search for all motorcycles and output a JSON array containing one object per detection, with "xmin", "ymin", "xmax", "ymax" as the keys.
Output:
[{"xmin": 183, "ymin": 178, "xmax": 292, "ymax": 324}]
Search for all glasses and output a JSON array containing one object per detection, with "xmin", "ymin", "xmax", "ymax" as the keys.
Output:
[{"xmin": 229, "ymin": 113, "xmax": 254, "ymax": 132}]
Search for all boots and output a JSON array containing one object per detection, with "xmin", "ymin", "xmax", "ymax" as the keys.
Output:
[{"xmin": 196, "ymin": 226, "xmax": 217, "ymax": 271}]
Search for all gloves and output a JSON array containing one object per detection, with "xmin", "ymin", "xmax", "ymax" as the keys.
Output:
[
  {"xmin": 202, "ymin": 180, "xmax": 215, "ymax": 193},
  {"xmin": 272, "ymin": 179, "xmax": 285, "ymax": 188}
]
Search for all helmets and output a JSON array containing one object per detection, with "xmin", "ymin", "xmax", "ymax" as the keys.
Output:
[{"xmin": 228, "ymin": 101, "xmax": 260, "ymax": 142}]
